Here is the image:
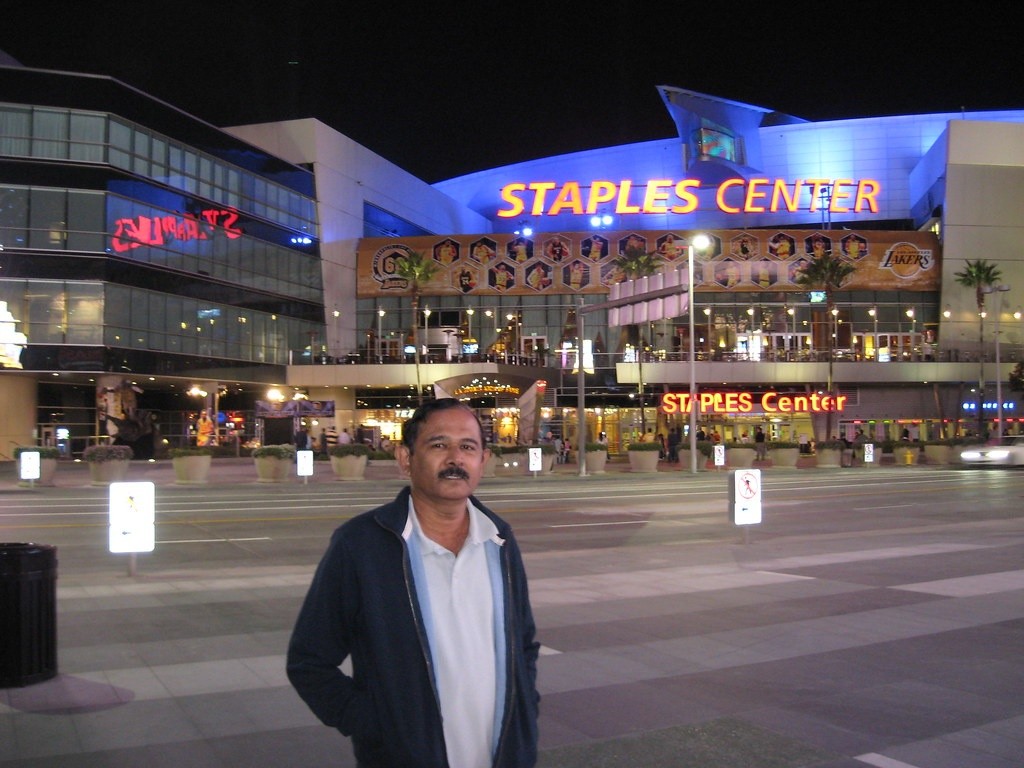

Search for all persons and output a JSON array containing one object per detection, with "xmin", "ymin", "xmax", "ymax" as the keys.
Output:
[
  {"xmin": 546, "ymin": 432, "xmax": 571, "ymax": 464},
  {"xmin": 755, "ymin": 427, "xmax": 766, "ymax": 461},
  {"xmin": 841, "ymin": 429, "xmax": 871, "ymax": 450},
  {"xmin": 293, "ymin": 425, "xmax": 311, "ymax": 464},
  {"xmin": 696, "ymin": 427, "xmax": 721, "ymax": 444},
  {"xmin": 493, "ymin": 428, "xmax": 512, "ymax": 444},
  {"xmin": 286, "ymin": 397, "xmax": 541, "ymax": 768},
  {"xmin": 196, "ymin": 409, "xmax": 215, "ymax": 447},
  {"xmin": 900, "ymin": 424, "xmax": 921, "ymax": 442},
  {"xmin": 357, "ymin": 423, "xmax": 365, "ymax": 444},
  {"xmin": 643, "ymin": 427, "xmax": 679, "ymax": 463},
  {"xmin": 434, "ymin": 234, "xmax": 865, "ymax": 293},
  {"xmin": 596, "ymin": 431, "xmax": 611, "ymax": 462},
  {"xmin": 320, "ymin": 426, "xmax": 350, "ymax": 454}
]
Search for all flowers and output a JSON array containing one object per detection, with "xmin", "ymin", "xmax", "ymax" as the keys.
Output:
[
  {"xmin": 169, "ymin": 447, "xmax": 217, "ymax": 458},
  {"xmin": 328, "ymin": 442, "xmax": 372, "ymax": 460},
  {"xmin": 84, "ymin": 444, "xmax": 136, "ymax": 464},
  {"xmin": 253, "ymin": 444, "xmax": 301, "ymax": 459},
  {"xmin": 16, "ymin": 444, "xmax": 62, "ymax": 459},
  {"xmin": 518, "ymin": 446, "xmax": 558, "ymax": 456},
  {"xmin": 485, "ymin": 442, "xmax": 503, "ymax": 455}
]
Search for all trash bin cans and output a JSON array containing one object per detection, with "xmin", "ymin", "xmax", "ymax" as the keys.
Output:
[{"xmin": 0, "ymin": 543, "xmax": 56, "ymax": 690}]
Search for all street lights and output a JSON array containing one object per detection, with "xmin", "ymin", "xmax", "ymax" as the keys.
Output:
[
  {"xmin": 905, "ymin": 307, "xmax": 917, "ymax": 362},
  {"xmin": 421, "ymin": 304, "xmax": 431, "ymax": 365},
  {"xmin": 831, "ymin": 307, "xmax": 838, "ymax": 361},
  {"xmin": 687, "ymin": 233, "xmax": 713, "ymax": 473},
  {"xmin": 484, "ymin": 304, "xmax": 498, "ymax": 363},
  {"xmin": 787, "ymin": 307, "xmax": 796, "ymax": 361},
  {"xmin": 375, "ymin": 305, "xmax": 386, "ymax": 365},
  {"xmin": 746, "ymin": 308, "xmax": 754, "ymax": 360},
  {"xmin": 703, "ymin": 307, "xmax": 712, "ymax": 362},
  {"xmin": 332, "ymin": 309, "xmax": 340, "ymax": 365},
  {"xmin": 466, "ymin": 304, "xmax": 475, "ymax": 363},
  {"xmin": 867, "ymin": 308, "xmax": 878, "ymax": 362},
  {"xmin": 980, "ymin": 282, "xmax": 1012, "ymax": 447},
  {"xmin": 507, "ymin": 309, "xmax": 519, "ymax": 367}
]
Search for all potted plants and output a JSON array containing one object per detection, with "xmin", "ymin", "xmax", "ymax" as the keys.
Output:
[
  {"xmin": 767, "ymin": 440, "xmax": 801, "ymax": 468},
  {"xmin": 852, "ymin": 435, "xmax": 885, "ymax": 468},
  {"xmin": 726, "ymin": 442, "xmax": 759, "ymax": 467},
  {"xmin": 575, "ymin": 443, "xmax": 610, "ymax": 474},
  {"xmin": 675, "ymin": 441, "xmax": 713, "ymax": 470},
  {"xmin": 813, "ymin": 438, "xmax": 847, "ymax": 466},
  {"xmin": 628, "ymin": 442, "xmax": 664, "ymax": 474},
  {"xmin": 893, "ymin": 438, "xmax": 992, "ymax": 465}
]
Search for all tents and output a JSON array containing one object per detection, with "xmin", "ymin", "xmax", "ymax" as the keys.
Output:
[{"xmin": 253, "ymin": 398, "xmax": 334, "ymax": 419}]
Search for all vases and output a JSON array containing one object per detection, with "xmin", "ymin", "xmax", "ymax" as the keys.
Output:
[
  {"xmin": 169, "ymin": 455, "xmax": 210, "ymax": 486},
  {"xmin": 253, "ymin": 456, "xmax": 289, "ymax": 483},
  {"xmin": 480, "ymin": 456, "xmax": 498, "ymax": 476},
  {"xmin": 89, "ymin": 455, "xmax": 128, "ymax": 486},
  {"xmin": 16, "ymin": 454, "xmax": 57, "ymax": 490},
  {"xmin": 522, "ymin": 454, "xmax": 551, "ymax": 475},
  {"xmin": 331, "ymin": 451, "xmax": 367, "ymax": 480}
]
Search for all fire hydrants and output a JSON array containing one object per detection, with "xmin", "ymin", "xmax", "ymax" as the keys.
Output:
[{"xmin": 903, "ymin": 449, "xmax": 915, "ymax": 466}]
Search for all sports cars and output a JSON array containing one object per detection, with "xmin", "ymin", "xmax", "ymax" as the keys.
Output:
[{"xmin": 948, "ymin": 438, "xmax": 1024, "ymax": 467}]
